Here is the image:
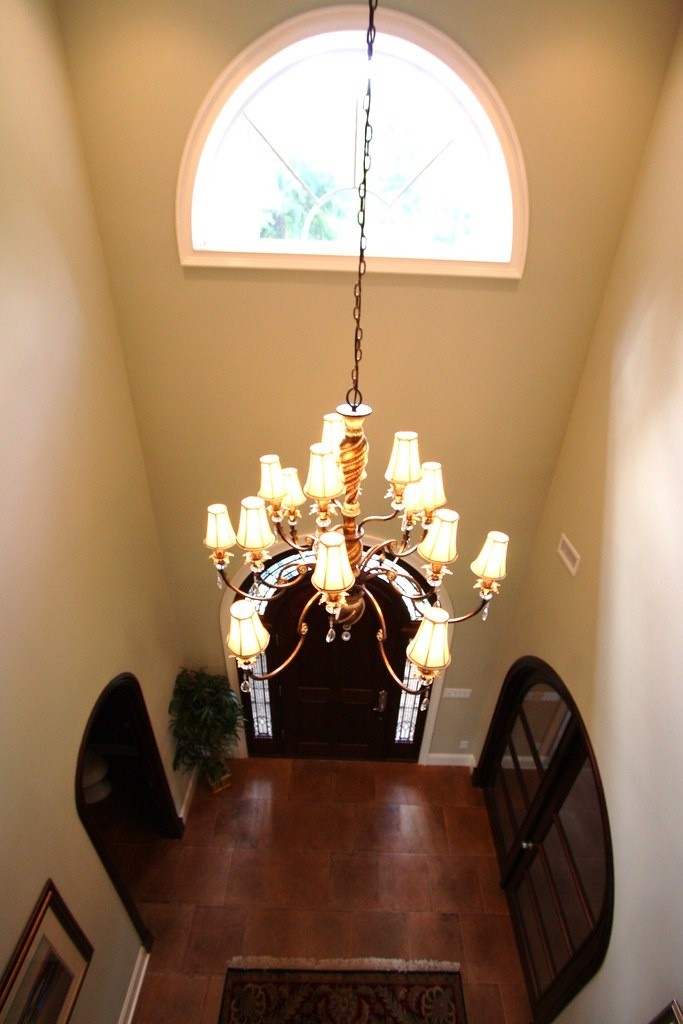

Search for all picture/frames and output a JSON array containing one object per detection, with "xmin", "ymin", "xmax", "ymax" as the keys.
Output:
[{"xmin": 0, "ymin": 878, "xmax": 94, "ymax": 1024}]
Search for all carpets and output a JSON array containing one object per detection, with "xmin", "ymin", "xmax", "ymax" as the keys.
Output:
[{"xmin": 214, "ymin": 950, "xmax": 469, "ymax": 1024}]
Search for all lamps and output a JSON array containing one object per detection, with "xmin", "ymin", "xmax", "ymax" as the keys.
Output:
[{"xmin": 199, "ymin": 402, "xmax": 512, "ymax": 713}]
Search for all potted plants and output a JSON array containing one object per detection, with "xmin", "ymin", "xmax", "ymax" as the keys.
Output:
[{"xmin": 166, "ymin": 665, "xmax": 245, "ymax": 794}]
